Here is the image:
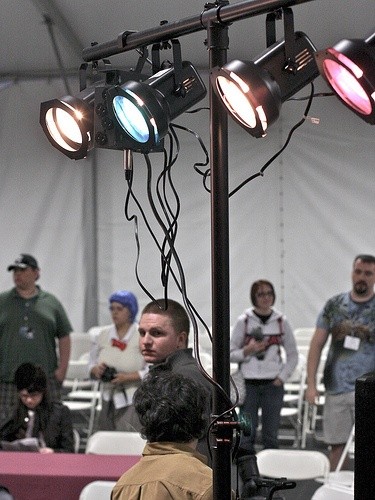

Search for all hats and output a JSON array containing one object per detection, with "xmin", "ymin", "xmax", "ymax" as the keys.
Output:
[
  {"xmin": 8, "ymin": 254, "xmax": 37, "ymax": 272},
  {"xmin": 108, "ymin": 290, "xmax": 138, "ymax": 323}
]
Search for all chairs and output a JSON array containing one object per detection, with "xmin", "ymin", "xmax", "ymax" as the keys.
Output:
[{"xmin": 65, "ymin": 327, "xmax": 356, "ymax": 500}]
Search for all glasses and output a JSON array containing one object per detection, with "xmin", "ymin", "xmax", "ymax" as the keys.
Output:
[{"xmin": 257, "ymin": 291, "xmax": 274, "ymax": 297}]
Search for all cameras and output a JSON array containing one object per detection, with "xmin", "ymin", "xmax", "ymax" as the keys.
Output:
[{"xmin": 101, "ymin": 368, "xmax": 117, "ymax": 382}]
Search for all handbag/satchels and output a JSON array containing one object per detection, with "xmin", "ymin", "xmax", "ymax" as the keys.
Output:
[{"xmin": 230, "ymin": 371, "xmax": 247, "ymax": 407}]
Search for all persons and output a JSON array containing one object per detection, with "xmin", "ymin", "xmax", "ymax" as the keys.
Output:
[
  {"xmin": 114, "ymin": 299, "xmax": 216, "ymax": 470},
  {"xmin": 230, "ymin": 279, "xmax": 298, "ymax": 465},
  {"xmin": 88, "ymin": 290, "xmax": 149, "ymax": 438},
  {"xmin": 0, "ymin": 254, "xmax": 73, "ymax": 421},
  {"xmin": 0, "ymin": 361, "xmax": 76, "ymax": 454},
  {"xmin": 306, "ymin": 254, "xmax": 375, "ymax": 472},
  {"xmin": 108, "ymin": 375, "xmax": 236, "ymax": 500}
]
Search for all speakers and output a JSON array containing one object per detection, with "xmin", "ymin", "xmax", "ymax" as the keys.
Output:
[{"xmin": 355, "ymin": 372, "xmax": 375, "ymax": 500}]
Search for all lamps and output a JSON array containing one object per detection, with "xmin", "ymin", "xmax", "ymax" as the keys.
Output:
[
  {"xmin": 39, "ymin": 57, "xmax": 120, "ymax": 161},
  {"xmin": 318, "ymin": 30, "xmax": 375, "ymax": 127},
  {"xmin": 209, "ymin": 8, "xmax": 322, "ymax": 138},
  {"xmin": 104, "ymin": 37, "xmax": 207, "ymax": 143}
]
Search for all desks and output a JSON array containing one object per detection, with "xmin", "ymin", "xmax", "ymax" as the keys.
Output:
[{"xmin": 0, "ymin": 450, "xmax": 140, "ymax": 499}]
